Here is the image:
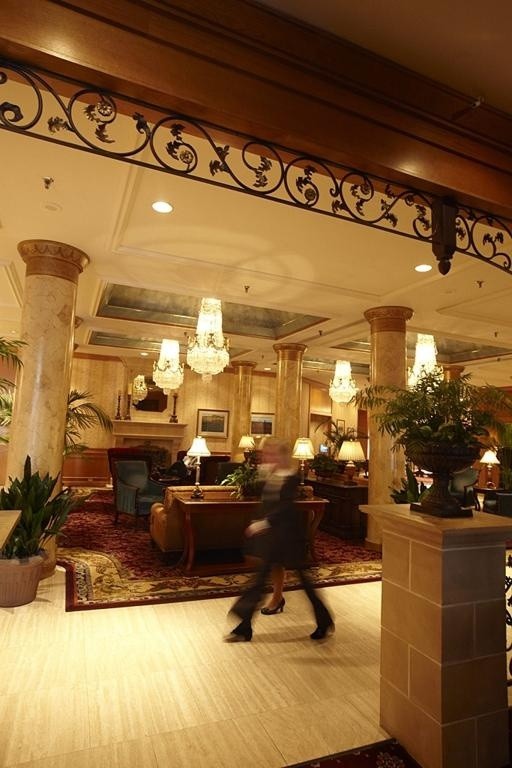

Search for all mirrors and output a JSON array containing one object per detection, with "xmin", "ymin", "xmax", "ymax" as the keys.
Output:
[{"xmin": 132, "ymin": 376, "xmax": 167, "ymax": 412}]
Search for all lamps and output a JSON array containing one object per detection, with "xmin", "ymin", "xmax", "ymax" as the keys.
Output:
[
  {"xmin": 238, "ymin": 436, "xmax": 256, "ymax": 463},
  {"xmin": 479, "ymin": 450, "xmax": 501, "ymax": 488},
  {"xmin": 327, "ymin": 361, "xmax": 358, "ymax": 403},
  {"xmin": 152, "ymin": 297, "xmax": 230, "ymax": 395},
  {"xmin": 408, "ymin": 333, "xmax": 444, "ymax": 387},
  {"xmin": 185, "ymin": 436, "xmax": 212, "ymax": 499},
  {"xmin": 292, "ymin": 438, "xmax": 366, "ymax": 485},
  {"xmin": 132, "ymin": 374, "xmax": 148, "ymax": 406}
]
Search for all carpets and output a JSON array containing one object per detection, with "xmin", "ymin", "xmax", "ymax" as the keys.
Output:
[
  {"xmin": 56, "ymin": 486, "xmax": 383, "ymax": 612},
  {"xmin": 281, "ymin": 737, "xmax": 421, "ymax": 768}
]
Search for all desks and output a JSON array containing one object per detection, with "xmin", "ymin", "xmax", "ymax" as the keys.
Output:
[
  {"xmin": 109, "ymin": 420, "xmax": 188, "ymax": 461},
  {"xmin": 174, "ymin": 495, "xmax": 330, "ymax": 577}
]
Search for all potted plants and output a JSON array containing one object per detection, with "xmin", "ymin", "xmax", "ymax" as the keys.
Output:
[
  {"xmin": 350, "ymin": 368, "xmax": 512, "ymax": 519},
  {"xmin": 0, "ymin": 454, "xmax": 97, "ymax": 607}
]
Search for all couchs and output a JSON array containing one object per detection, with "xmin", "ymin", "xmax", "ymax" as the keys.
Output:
[{"xmin": 150, "ymin": 484, "xmax": 313, "ymax": 553}]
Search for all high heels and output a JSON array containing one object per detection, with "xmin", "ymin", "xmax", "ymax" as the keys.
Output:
[
  {"xmin": 310, "ymin": 622, "xmax": 336, "ymax": 639},
  {"xmin": 225, "ymin": 627, "xmax": 252, "ymax": 641},
  {"xmin": 261, "ymin": 599, "xmax": 285, "ymax": 614}
]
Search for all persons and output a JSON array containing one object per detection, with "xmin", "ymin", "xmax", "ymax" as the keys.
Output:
[
  {"xmin": 250, "ymin": 437, "xmax": 286, "ymax": 617},
  {"xmin": 229, "ymin": 446, "xmax": 335, "ymax": 642}
]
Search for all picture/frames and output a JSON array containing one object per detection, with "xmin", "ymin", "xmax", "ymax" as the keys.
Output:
[
  {"xmin": 250, "ymin": 412, "xmax": 276, "ymax": 437},
  {"xmin": 197, "ymin": 409, "xmax": 230, "ymax": 439}
]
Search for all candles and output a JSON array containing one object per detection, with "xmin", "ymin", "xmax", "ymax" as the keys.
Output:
[{"xmin": 127, "ymin": 381, "xmax": 133, "ymax": 395}]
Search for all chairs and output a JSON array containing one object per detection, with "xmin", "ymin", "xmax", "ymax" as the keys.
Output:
[
  {"xmin": 449, "ymin": 466, "xmax": 511, "ymax": 517},
  {"xmin": 108, "ymin": 458, "xmax": 265, "ymax": 531}
]
